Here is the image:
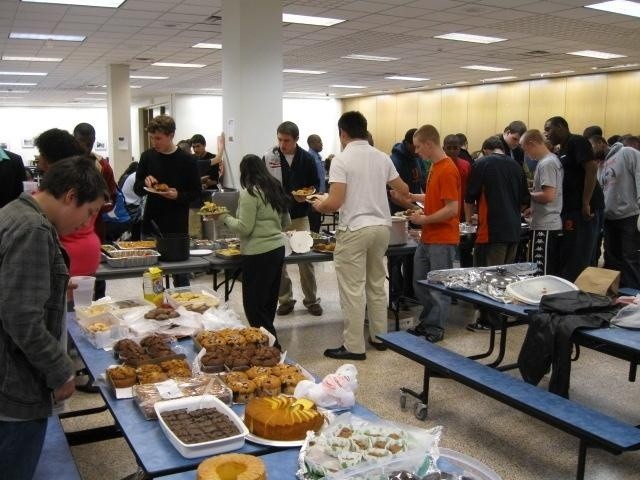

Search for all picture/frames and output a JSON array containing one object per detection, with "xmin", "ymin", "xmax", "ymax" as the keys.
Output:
[
  {"xmin": 22, "ymin": 137, "xmax": 35, "ymax": 148},
  {"xmin": 95, "ymin": 140, "xmax": 107, "ymax": 150}
]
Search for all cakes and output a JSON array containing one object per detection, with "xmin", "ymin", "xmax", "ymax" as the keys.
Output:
[
  {"xmin": 197, "ymin": 453, "xmax": 266, "ymax": 480},
  {"xmin": 107, "ymin": 328, "xmax": 324, "ymax": 444},
  {"xmin": 328, "ymin": 423, "xmax": 406, "ymax": 465}
]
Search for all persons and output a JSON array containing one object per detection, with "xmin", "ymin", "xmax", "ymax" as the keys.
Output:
[
  {"xmin": 1, "ymin": 154, "xmax": 111, "ymax": 480},
  {"xmin": 73, "ymin": 121, "xmax": 225, "ymax": 244},
  {"xmin": 260, "ymin": 120, "xmax": 323, "ymax": 316},
  {"xmin": 133, "ymin": 115, "xmax": 203, "ymax": 289},
  {"xmin": 32, "ymin": 128, "xmax": 104, "ymax": 279},
  {"xmin": 206, "ymin": 153, "xmax": 292, "ymax": 354},
  {"xmin": 390, "ymin": 116, "xmax": 640, "ymax": 343},
  {"xmin": 306, "ymin": 134, "xmax": 326, "ymax": 235},
  {"xmin": 0, "ymin": 144, "xmax": 28, "ymax": 208},
  {"xmin": 310, "ymin": 110, "xmax": 411, "ymax": 359}
]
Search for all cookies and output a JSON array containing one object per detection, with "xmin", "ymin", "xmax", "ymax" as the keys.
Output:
[
  {"xmin": 82, "ymin": 300, "xmax": 142, "ymax": 333},
  {"xmin": 169, "ymin": 291, "xmax": 218, "ymax": 312}
]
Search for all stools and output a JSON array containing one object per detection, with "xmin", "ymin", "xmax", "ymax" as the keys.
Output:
[{"xmin": 616, "ymin": 287, "xmax": 639, "ymax": 296}]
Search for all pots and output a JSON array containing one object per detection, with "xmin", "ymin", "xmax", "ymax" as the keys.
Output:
[{"xmin": 388, "ymin": 215, "xmax": 410, "ymax": 246}]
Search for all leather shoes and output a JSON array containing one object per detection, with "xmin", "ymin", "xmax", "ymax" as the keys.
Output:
[
  {"xmin": 368, "ymin": 335, "xmax": 388, "ymax": 351},
  {"xmin": 324, "ymin": 345, "xmax": 366, "ymax": 360}
]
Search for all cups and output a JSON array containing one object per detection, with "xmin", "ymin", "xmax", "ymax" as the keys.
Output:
[{"xmin": 71, "ymin": 275, "xmax": 96, "ymax": 310}]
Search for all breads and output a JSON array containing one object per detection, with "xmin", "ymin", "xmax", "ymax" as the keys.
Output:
[
  {"xmin": 153, "ymin": 182, "xmax": 169, "ymax": 191},
  {"xmin": 313, "ymin": 243, "xmax": 335, "ymax": 250},
  {"xmin": 145, "ymin": 302, "xmax": 179, "ymax": 320}
]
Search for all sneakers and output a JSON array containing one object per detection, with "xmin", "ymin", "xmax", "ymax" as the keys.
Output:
[
  {"xmin": 466, "ymin": 322, "xmax": 502, "ymax": 334},
  {"xmin": 308, "ymin": 304, "xmax": 322, "ymax": 315},
  {"xmin": 277, "ymin": 300, "xmax": 294, "ymax": 315},
  {"xmin": 406, "ymin": 323, "xmax": 444, "ymax": 343}
]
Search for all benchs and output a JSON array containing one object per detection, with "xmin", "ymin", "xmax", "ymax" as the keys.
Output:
[
  {"xmin": 375, "ymin": 330, "xmax": 639, "ymax": 480},
  {"xmin": 34, "ymin": 412, "xmax": 85, "ymax": 479}
]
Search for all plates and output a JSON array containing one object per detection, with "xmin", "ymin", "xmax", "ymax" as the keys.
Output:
[
  {"xmin": 293, "ymin": 188, "xmax": 317, "ymax": 196},
  {"xmin": 315, "ymin": 248, "xmax": 333, "ymax": 255},
  {"xmin": 305, "ymin": 199, "xmax": 318, "ymax": 204},
  {"xmin": 286, "ymin": 229, "xmax": 314, "ymax": 254},
  {"xmin": 143, "ymin": 187, "xmax": 173, "ymax": 196},
  {"xmin": 197, "ymin": 210, "xmax": 233, "ymax": 216},
  {"xmin": 189, "ymin": 248, "xmax": 214, "ymax": 256}
]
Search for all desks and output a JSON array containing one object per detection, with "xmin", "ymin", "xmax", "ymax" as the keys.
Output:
[
  {"xmin": 64, "ymin": 310, "xmax": 459, "ymax": 480},
  {"xmin": 90, "ymin": 220, "xmax": 533, "ymax": 301}
]
